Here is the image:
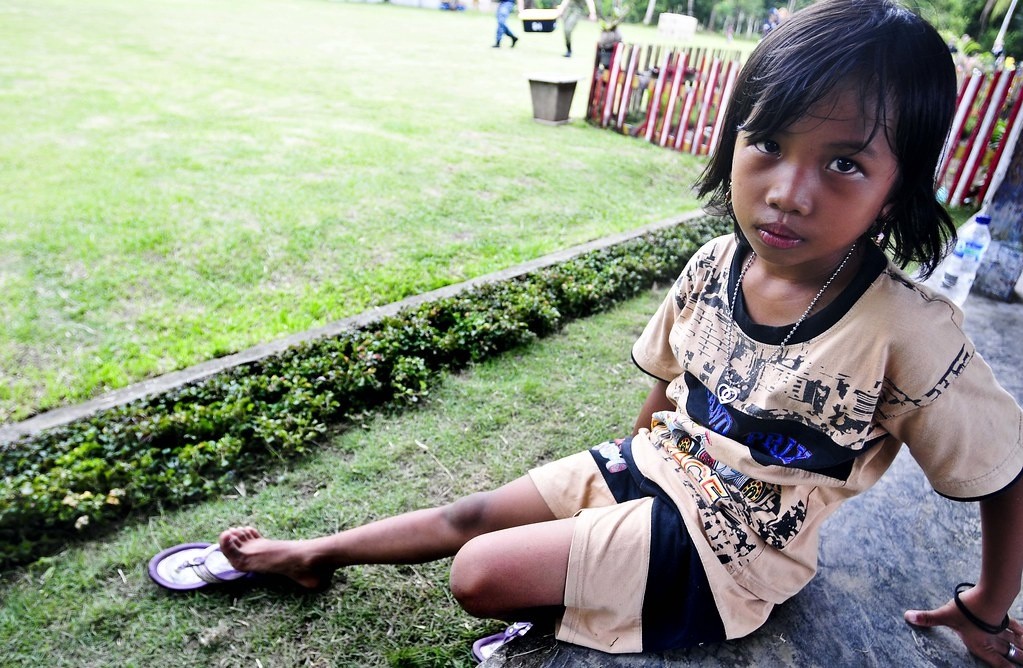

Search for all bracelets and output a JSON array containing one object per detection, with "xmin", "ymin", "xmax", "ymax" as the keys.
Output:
[{"xmin": 954, "ymin": 583, "xmax": 1010, "ymax": 635}]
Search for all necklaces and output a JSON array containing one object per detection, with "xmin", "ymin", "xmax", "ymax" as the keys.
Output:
[{"xmin": 717, "ymin": 242, "xmax": 856, "ymax": 404}]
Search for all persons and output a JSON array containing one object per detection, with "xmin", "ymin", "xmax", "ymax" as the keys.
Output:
[
  {"xmin": 492, "ymin": 0, "xmax": 518, "ymax": 48},
  {"xmin": 559, "ymin": 0, "xmax": 597, "ymax": 58},
  {"xmin": 218, "ymin": 0, "xmax": 1023, "ymax": 668}
]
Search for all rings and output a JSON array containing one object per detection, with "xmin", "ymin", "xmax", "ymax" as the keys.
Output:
[{"xmin": 1008, "ymin": 643, "xmax": 1015, "ymax": 658}]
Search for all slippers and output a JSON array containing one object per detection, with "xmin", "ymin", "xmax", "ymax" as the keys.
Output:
[
  {"xmin": 472, "ymin": 618, "xmax": 533, "ymax": 663},
  {"xmin": 147, "ymin": 541, "xmax": 280, "ymax": 592}
]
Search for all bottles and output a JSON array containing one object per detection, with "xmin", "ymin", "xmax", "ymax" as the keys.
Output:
[{"xmin": 936, "ymin": 215, "xmax": 993, "ymax": 311}]
